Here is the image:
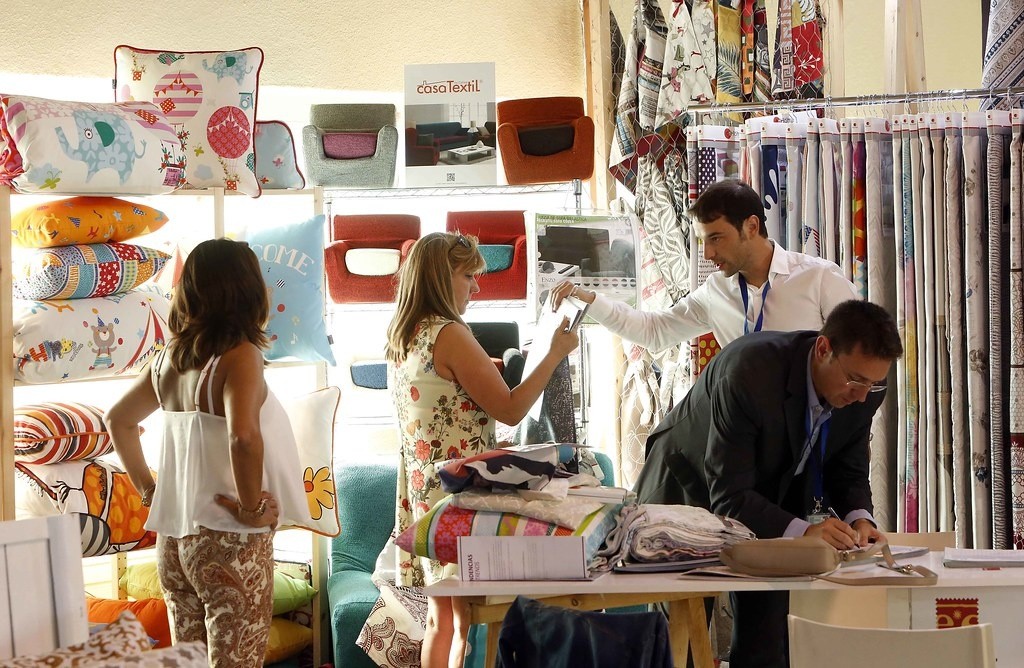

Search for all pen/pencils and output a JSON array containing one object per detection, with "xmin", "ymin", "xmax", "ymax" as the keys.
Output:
[{"xmin": 828, "ymin": 507, "xmax": 860, "ymax": 549}]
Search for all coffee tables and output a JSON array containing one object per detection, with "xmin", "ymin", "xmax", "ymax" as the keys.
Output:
[{"xmin": 448, "ymin": 145, "xmax": 495, "ymax": 165}]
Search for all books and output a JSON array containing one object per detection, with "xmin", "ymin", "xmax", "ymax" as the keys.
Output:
[
  {"xmin": 943, "ymin": 546, "xmax": 1024, "ymax": 568},
  {"xmin": 841, "ymin": 543, "xmax": 929, "ymax": 566}
]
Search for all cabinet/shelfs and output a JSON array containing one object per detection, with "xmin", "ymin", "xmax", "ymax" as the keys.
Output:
[
  {"xmin": 0, "ymin": 184, "xmax": 332, "ymax": 668},
  {"xmin": 422, "ymin": 549, "xmax": 1024, "ymax": 668},
  {"xmin": 319, "ymin": 179, "xmax": 588, "ymax": 452}
]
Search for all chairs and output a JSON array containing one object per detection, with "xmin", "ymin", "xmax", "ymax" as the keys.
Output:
[{"xmin": 788, "ymin": 615, "xmax": 993, "ymax": 668}]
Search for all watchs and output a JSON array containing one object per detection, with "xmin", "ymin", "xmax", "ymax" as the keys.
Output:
[{"xmin": 239, "ymin": 499, "xmax": 267, "ymax": 519}]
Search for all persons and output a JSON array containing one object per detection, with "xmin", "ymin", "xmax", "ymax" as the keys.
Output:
[
  {"xmin": 550, "ymin": 179, "xmax": 858, "ymax": 354},
  {"xmin": 384, "ymin": 231, "xmax": 580, "ymax": 668},
  {"xmin": 632, "ymin": 300, "xmax": 904, "ymax": 668},
  {"xmin": 102, "ymin": 238, "xmax": 311, "ymax": 668}
]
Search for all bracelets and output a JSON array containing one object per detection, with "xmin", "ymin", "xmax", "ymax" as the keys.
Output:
[{"xmin": 142, "ymin": 484, "xmax": 156, "ymax": 507}]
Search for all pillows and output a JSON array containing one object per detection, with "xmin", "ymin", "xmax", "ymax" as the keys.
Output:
[
  {"xmin": 0, "ymin": 45, "xmax": 340, "ymax": 668},
  {"xmin": 393, "ymin": 491, "xmax": 631, "ymax": 566},
  {"xmin": 418, "ymin": 133, "xmax": 434, "ymax": 147},
  {"xmin": 476, "ymin": 127, "xmax": 490, "ymax": 135}
]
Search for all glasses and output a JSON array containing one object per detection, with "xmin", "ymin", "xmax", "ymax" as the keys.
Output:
[
  {"xmin": 448, "ymin": 234, "xmax": 472, "ymax": 252},
  {"xmin": 827, "ymin": 344, "xmax": 888, "ymax": 392}
]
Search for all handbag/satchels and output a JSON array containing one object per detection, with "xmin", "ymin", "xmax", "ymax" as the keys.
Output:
[{"xmin": 717, "ymin": 536, "xmax": 845, "ymax": 581}]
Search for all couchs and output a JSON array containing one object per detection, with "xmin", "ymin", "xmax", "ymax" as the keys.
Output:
[
  {"xmin": 416, "ymin": 121, "xmax": 473, "ymax": 151},
  {"xmin": 474, "ymin": 121, "xmax": 496, "ymax": 149},
  {"xmin": 326, "ymin": 451, "xmax": 614, "ymax": 668},
  {"xmin": 405, "ymin": 128, "xmax": 439, "ymax": 166},
  {"xmin": 302, "ymin": 96, "xmax": 595, "ymax": 417}
]
